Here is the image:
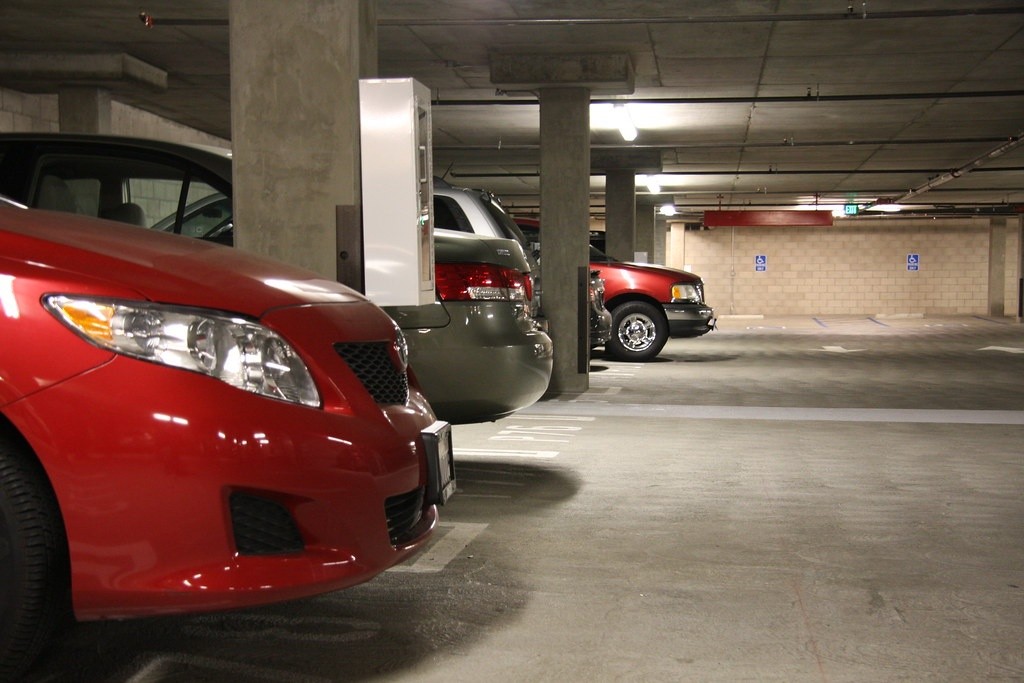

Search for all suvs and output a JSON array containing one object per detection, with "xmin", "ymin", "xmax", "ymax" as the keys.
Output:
[
  {"xmin": 150, "ymin": 172, "xmax": 612, "ymax": 425},
  {"xmin": 511, "ymin": 212, "xmax": 718, "ymax": 361}
]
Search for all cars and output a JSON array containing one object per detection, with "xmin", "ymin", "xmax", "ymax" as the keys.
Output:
[
  {"xmin": 0, "ymin": 127, "xmax": 236, "ymax": 248},
  {"xmin": 0, "ymin": 196, "xmax": 459, "ymax": 682}
]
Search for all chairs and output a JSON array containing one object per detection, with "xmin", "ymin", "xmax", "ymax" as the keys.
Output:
[{"xmin": 110, "ymin": 202, "xmax": 146, "ymax": 227}]
